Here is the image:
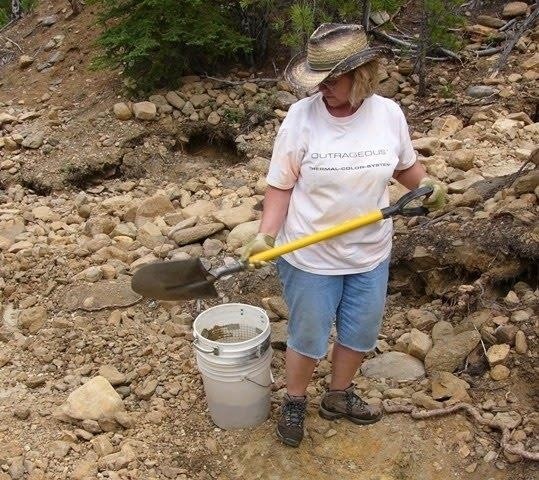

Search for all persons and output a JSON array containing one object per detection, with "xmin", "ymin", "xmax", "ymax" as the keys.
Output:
[{"xmin": 238, "ymin": 21, "xmax": 447, "ymax": 449}]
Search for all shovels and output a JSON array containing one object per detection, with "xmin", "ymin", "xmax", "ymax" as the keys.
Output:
[{"xmin": 132, "ymin": 184, "xmax": 434, "ymax": 301}]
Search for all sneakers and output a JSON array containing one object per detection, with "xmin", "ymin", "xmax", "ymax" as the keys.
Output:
[
  {"xmin": 319, "ymin": 383, "xmax": 380, "ymax": 425},
  {"xmin": 276, "ymin": 394, "xmax": 306, "ymax": 447}
]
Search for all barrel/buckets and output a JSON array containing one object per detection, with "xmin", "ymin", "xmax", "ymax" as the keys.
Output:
[{"xmin": 192, "ymin": 303, "xmax": 275, "ymax": 431}]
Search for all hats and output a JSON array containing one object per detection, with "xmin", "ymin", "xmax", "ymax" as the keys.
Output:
[{"xmin": 284, "ymin": 23, "xmax": 395, "ymax": 92}]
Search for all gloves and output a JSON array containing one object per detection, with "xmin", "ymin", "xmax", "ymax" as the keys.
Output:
[
  {"xmin": 241, "ymin": 233, "xmax": 275, "ymax": 271},
  {"xmin": 418, "ymin": 178, "xmax": 448, "ymax": 208}
]
